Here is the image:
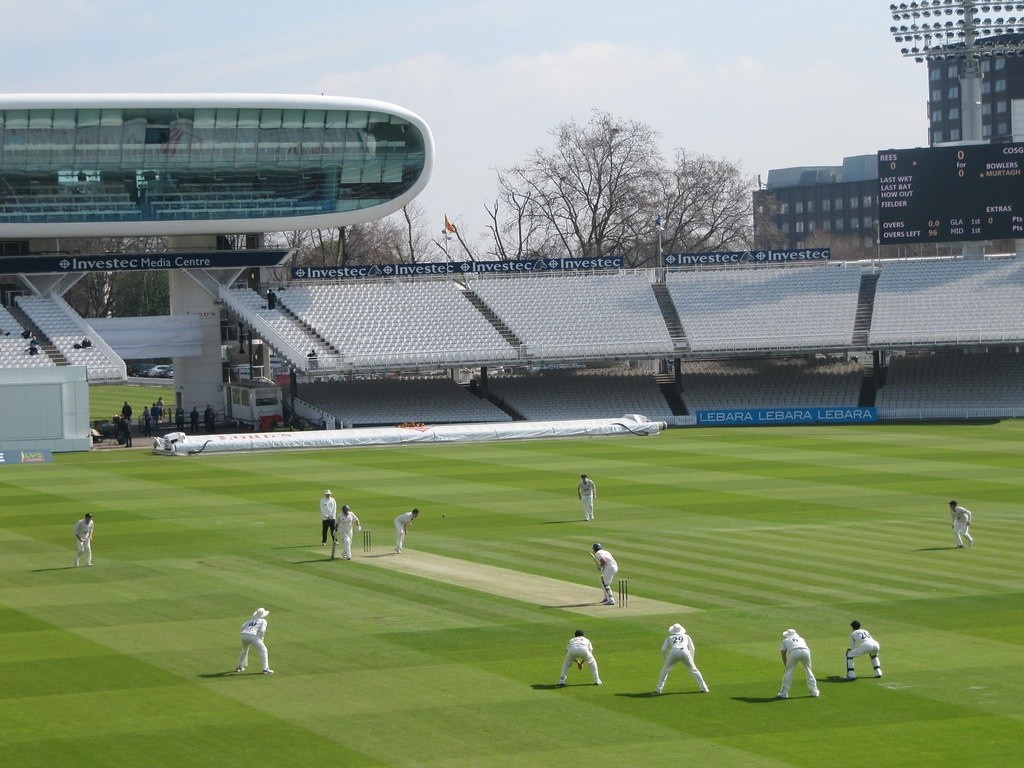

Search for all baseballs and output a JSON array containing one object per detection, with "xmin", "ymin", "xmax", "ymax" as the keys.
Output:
[{"xmin": 442, "ymin": 514, "xmax": 445, "ymax": 517}]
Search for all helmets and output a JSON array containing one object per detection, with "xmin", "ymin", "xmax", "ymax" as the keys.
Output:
[
  {"xmin": 593, "ymin": 543, "xmax": 601, "ymax": 550},
  {"xmin": 342, "ymin": 505, "xmax": 349, "ymax": 510}
]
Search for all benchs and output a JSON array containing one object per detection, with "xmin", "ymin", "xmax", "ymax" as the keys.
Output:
[{"xmin": 1, "ymin": 176, "xmax": 322, "ymax": 221}]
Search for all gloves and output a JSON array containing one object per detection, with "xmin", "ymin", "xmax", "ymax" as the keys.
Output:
[
  {"xmin": 357, "ymin": 525, "xmax": 361, "ymax": 531},
  {"xmin": 333, "ymin": 530, "xmax": 337, "ymax": 537}
]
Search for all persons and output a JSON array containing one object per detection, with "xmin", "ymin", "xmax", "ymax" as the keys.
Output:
[
  {"xmin": 394, "ymin": 508, "xmax": 419, "ymax": 553},
  {"xmin": 333, "ymin": 505, "xmax": 362, "ymax": 560},
  {"xmin": 236, "ymin": 608, "xmax": 274, "ymax": 674},
  {"xmin": 776, "ymin": 629, "xmax": 820, "ymax": 698},
  {"xmin": 81, "ymin": 337, "xmax": 92, "ymax": 348},
  {"xmin": 320, "ymin": 490, "xmax": 340, "ymax": 546},
  {"xmin": 949, "ymin": 501, "xmax": 973, "ymax": 548},
  {"xmin": 75, "ymin": 513, "xmax": 94, "ymax": 566},
  {"xmin": 841, "ymin": 620, "xmax": 882, "ymax": 680},
  {"xmin": 266, "ymin": 289, "xmax": 277, "ymax": 310},
  {"xmin": 308, "ymin": 351, "xmax": 317, "ymax": 369},
  {"xmin": 652, "ymin": 623, "xmax": 709, "ymax": 693},
  {"xmin": 590, "ymin": 543, "xmax": 618, "ymax": 605},
  {"xmin": 116, "ymin": 396, "xmax": 216, "ymax": 448},
  {"xmin": 578, "ymin": 474, "xmax": 596, "ymax": 521},
  {"xmin": 558, "ymin": 630, "xmax": 602, "ymax": 686},
  {"xmin": 21, "ymin": 326, "xmax": 40, "ymax": 356}
]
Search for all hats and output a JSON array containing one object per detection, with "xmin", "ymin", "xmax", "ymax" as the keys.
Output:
[
  {"xmin": 325, "ymin": 490, "xmax": 332, "ymax": 494},
  {"xmin": 669, "ymin": 623, "xmax": 686, "ymax": 635},
  {"xmin": 253, "ymin": 608, "xmax": 269, "ymax": 619},
  {"xmin": 86, "ymin": 513, "xmax": 93, "ymax": 517},
  {"xmin": 783, "ymin": 629, "xmax": 799, "ymax": 637},
  {"xmin": 949, "ymin": 501, "xmax": 956, "ymax": 505},
  {"xmin": 575, "ymin": 629, "xmax": 584, "ymax": 636},
  {"xmin": 850, "ymin": 621, "xmax": 860, "ymax": 628}
]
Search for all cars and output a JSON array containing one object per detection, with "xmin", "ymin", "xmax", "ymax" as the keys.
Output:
[{"xmin": 130, "ymin": 363, "xmax": 173, "ymax": 377}]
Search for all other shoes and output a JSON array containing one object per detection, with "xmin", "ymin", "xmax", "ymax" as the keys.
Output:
[
  {"xmin": 876, "ymin": 672, "xmax": 883, "ymax": 676},
  {"xmin": 655, "ymin": 688, "xmax": 662, "ymax": 694},
  {"xmin": 585, "ymin": 516, "xmax": 594, "ymax": 521},
  {"xmin": 595, "ymin": 679, "xmax": 602, "ymax": 684},
  {"xmin": 342, "ymin": 553, "xmax": 351, "ymax": 560},
  {"xmin": 396, "ymin": 547, "xmax": 402, "ymax": 554},
  {"xmin": 843, "ymin": 675, "xmax": 855, "ymax": 680},
  {"xmin": 701, "ymin": 688, "xmax": 709, "ymax": 693},
  {"xmin": 601, "ymin": 598, "xmax": 615, "ymax": 605},
  {"xmin": 559, "ymin": 679, "xmax": 565, "ymax": 685},
  {"xmin": 335, "ymin": 541, "xmax": 339, "ymax": 545},
  {"xmin": 813, "ymin": 689, "xmax": 819, "ymax": 697},
  {"xmin": 777, "ymin": 692, "xmax": 788, "ymax": 699},
  {"xmin": 323, "ymin": 542, "xmax": 326, "ymax": 546},
  {"xmin": 263, "ymin": 668, "xmax": 274, "ymax": 674},
  {"xmin": 236, "ymin": 666, "xmax": 245, "ymax": 672}
]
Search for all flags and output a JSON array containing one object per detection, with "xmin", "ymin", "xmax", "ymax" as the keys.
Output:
[
  {"xmin": 444, "ymin": 215, "xmax": 456, "ymax": 233},
  {"xmin": 168, "ymin": 127, "xmax": 183, "ymax": 151},
  {"xmin": 656, "ymin": 213, "xmax": 662, "ymax": 226}
]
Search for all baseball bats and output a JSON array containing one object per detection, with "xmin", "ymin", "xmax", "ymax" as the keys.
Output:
[
  {"xmin": 587, "ymin": 550, "xmax": 603, "ymax": 573},
  {"xmin": 331, "ymin": 523, "xmax": 338, "ymax": 559}
]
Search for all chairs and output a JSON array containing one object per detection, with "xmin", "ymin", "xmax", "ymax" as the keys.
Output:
[
  {"xmin": 219, "ymin": 255, "xmax": 1024, "ymax": 429},
  {"xmin": 0, "ymin": 295, "xmax": 122, "ymax": 380}
]
